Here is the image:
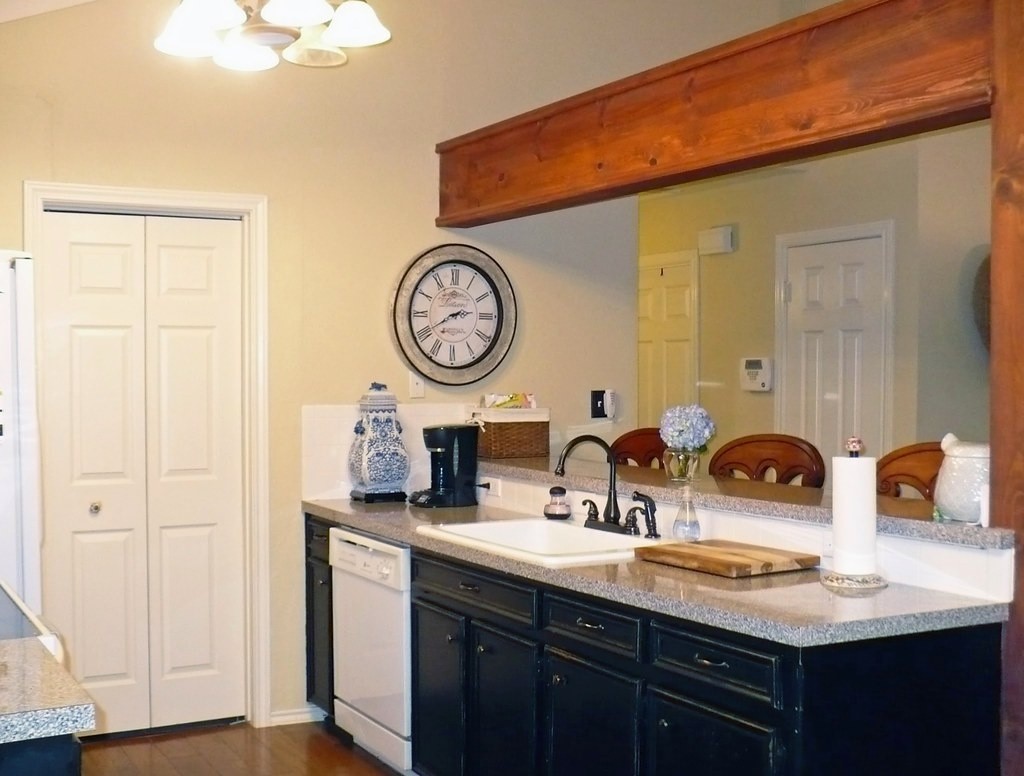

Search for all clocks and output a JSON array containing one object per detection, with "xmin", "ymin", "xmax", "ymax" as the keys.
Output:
[{"xmin": 393, "ymin": 241, "xmax": 519, "ymax": 388}]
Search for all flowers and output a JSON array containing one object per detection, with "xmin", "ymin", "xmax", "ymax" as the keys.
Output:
[{"xmin": 659, "ymin": 401, "xmax": 720, "ymax": 453}]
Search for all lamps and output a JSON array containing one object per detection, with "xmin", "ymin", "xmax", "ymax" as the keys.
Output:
[{"xmin": 151, "ymin": 0, "xmax": 394, "ymax": 76}]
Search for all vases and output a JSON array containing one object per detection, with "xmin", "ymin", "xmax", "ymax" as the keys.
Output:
[{"xmin": 662, "ymin": 447, "xmax": 700, "ymax": 484}]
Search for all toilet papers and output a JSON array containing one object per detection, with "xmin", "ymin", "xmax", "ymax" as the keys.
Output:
[{"xmin": 830, "ymin": 456, "xmax": 879, "ymax": 576}]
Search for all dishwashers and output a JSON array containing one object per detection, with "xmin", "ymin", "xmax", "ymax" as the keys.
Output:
[{"xmin": 329, "ymin": 526, "xmax": 412, "ymax": 772}]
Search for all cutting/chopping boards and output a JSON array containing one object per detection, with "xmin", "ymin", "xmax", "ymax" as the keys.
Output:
[{"xmin": 634, "ymin": 538, "xmax": 821, "ymax": 578}]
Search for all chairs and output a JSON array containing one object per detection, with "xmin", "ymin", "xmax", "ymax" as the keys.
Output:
[
  {"xmin": 876, "ymin": 438, "xmax": 945, "ymax": 501},
  {"xmin": 709, "ymin": 432, "xmax": 827, "ymax": 489},
  {"xmin": 607, "ymin": 427, "xmax": 666, "ymax": 469}
]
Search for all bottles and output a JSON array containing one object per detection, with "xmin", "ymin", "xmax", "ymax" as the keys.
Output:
[
  {"xmin": 545, "ymin": 486, "xmax": 572, "ymax": 519},
  {"xmin": 935, "ymin": 433, "xmax": 989, "ymax": 524},
  {"xmin": 346, "ymin": 384, "xmax": 409, "ymax": 490}
]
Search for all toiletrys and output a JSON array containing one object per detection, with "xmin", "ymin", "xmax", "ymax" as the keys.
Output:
[{"xmin": 544, "ymin": 486, "xmax": 571, "ymax": 519}]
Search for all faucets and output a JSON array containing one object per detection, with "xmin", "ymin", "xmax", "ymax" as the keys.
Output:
[{"xmin": 553, "ymin": 434, "xmax": 646, "ymax": 536}]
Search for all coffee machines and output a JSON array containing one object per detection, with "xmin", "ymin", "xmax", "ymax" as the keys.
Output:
[{"xmin": 410, "ymin": 424, "xmax": 479, "ymax": 510}]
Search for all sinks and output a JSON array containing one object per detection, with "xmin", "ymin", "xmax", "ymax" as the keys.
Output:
[{"xmin": 417, "ymin": 517, "xmax": 677, "ymax": 571}]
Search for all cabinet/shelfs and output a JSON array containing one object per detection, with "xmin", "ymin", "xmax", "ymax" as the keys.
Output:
[{"xmin": 298, "ymin": 517, "xmax": 1007, "ymax": 776}]
